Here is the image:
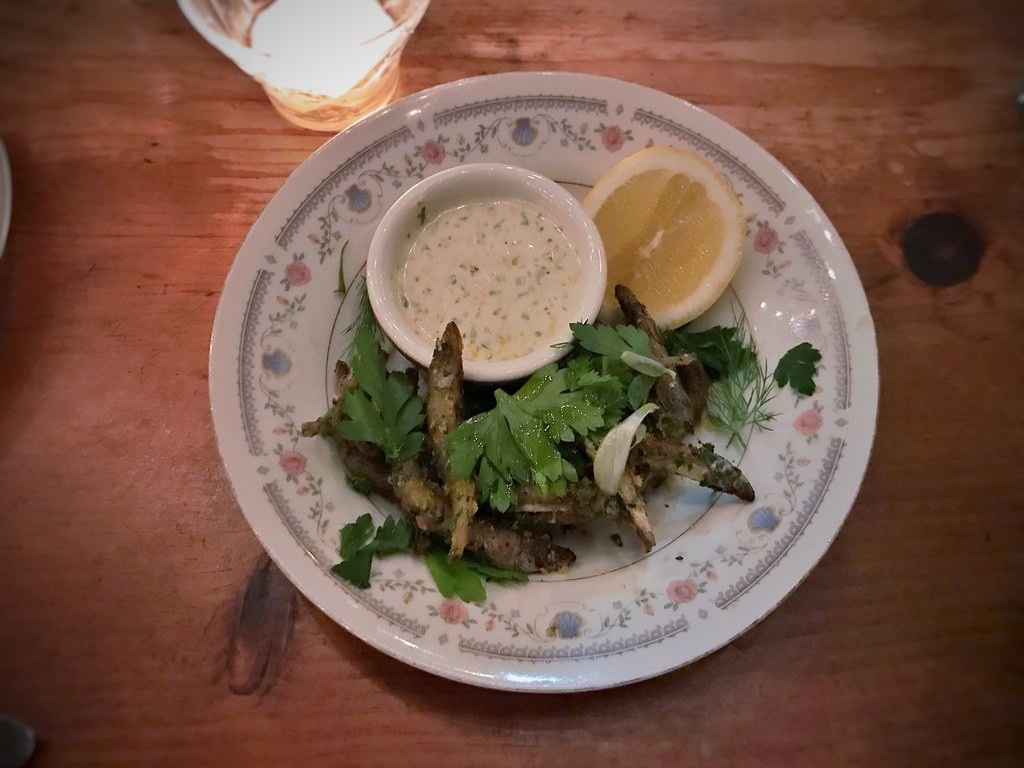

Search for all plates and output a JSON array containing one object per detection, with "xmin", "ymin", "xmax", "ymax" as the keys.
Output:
[
  {"xmin": 209, "ymin": 72, "xmax": 881, "ymax": 696},
  {"xmin": 0, "ymin": 140, "xmax": 12, "ymax": 258}
]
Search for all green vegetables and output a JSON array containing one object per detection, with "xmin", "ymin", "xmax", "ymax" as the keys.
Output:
[{"xmin": 321, "ymin": 239, "xmax": 821, "ymax": 604}]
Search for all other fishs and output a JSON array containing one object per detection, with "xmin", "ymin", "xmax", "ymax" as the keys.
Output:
[{"xmin": 300, "ymin": 283, "xmax": 756, "ymax": 575}]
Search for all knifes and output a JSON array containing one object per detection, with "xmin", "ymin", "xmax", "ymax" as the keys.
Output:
[{"xmin": 225, "ymin": 548, "xmax": 292, "ymax": 696}]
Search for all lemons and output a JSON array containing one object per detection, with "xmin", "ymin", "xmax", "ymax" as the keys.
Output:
[{"xmin": 577, "ymin": 144, "xmax": 747, "ymax": 333}]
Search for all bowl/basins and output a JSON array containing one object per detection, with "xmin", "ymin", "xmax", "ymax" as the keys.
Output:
[{"xmin": 366, "ymin": 165, "xmax": 607, "ymax": 383}]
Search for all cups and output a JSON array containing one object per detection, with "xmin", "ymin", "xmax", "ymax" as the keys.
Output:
[{"xmin": 176, "ymin": 0, "xmax": 432, "ymax": 132}]
